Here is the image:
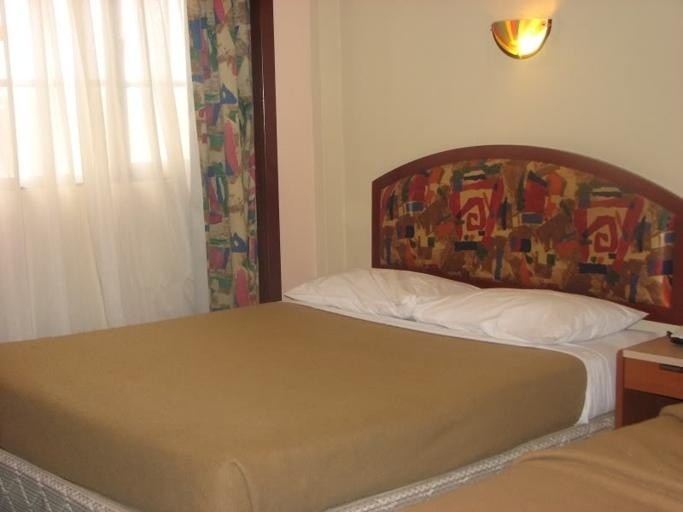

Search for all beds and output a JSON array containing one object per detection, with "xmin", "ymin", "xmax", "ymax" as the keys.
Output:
[{"xmin": 1, "ymin": 143, "xmax": 683, "ymax": 511}]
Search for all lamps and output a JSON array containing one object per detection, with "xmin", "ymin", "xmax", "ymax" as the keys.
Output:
[{"xmin": 490, "ymin": 17, "xmax": 552, "ymax": 60}]
